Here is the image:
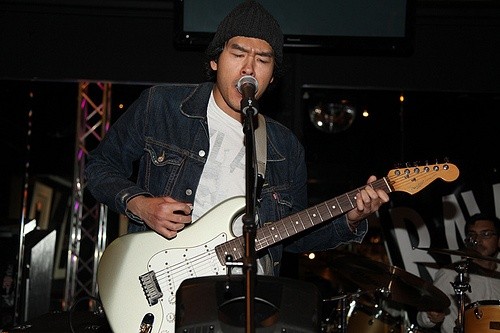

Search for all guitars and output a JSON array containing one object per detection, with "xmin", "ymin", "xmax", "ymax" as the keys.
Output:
[{"xmin": 97, "ymin": 163, "xmax": 460, "ymax": 332}]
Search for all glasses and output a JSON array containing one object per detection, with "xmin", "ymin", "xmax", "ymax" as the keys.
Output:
[{"xmin": 467, "ymin": 231, "xmax": 498, "ymax": 239}]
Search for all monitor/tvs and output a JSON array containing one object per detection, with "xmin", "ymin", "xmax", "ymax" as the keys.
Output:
[{"xmin": 173, "ymin": 0, "xmax": 409, "ymax": 56}]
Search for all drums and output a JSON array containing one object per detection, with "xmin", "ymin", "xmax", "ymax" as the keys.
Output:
[
  {"xmin": 347, "ymin": 302, "xmax": 401, "ymax": 332},
  {"xmin": 465, "ymin": 299, "xmax": 500, "ymax": 333}
]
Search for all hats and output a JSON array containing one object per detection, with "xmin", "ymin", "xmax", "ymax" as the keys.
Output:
[{"xmin": 207, "ymin": 0, "xmax": 283, "ymax": 69}]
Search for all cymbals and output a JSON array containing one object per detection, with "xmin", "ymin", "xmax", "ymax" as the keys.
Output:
[
  {"xmin": 414, "ymin": 261, "xmax": 500, "ymax": 279},
  {"xmin": 304, "ymin": 249, "xmax": 374, "ymax": 281},
  {"xmin": 322, "ymin": 254, "xmax": 451, "ymax": 312},
  {"xmin": 414, "ymin": 246, "xmax": 500, "ymax": 262}
]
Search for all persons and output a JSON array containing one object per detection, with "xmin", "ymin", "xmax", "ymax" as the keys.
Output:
[
  {"xmin": 84, "ymin": 3, "xmax": 391, "ymax": 278},
  {"xmin": 417, "ymin": 213, "xmax": 500, "ymax": 333}
]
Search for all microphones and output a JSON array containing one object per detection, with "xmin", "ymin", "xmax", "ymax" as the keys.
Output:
[{"xmin": 239, "ymin": 75, "xmax": 259, "ymax": 117}]
